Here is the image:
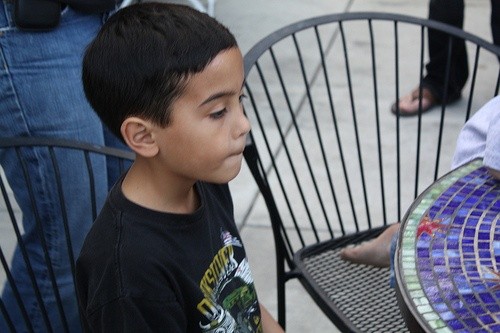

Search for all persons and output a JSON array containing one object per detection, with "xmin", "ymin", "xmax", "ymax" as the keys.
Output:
[
  {"xmin": 339, "ymin": 93, "xmax": 500, "ymax": 267},
  {"xmin": 74, "ymin": 0, "xmax": 288, "ymax": 333},
  {"xmin": 391, "ymin": 0, "xmax": 500, "ymax": 116},
  {"xmin": 0, "ymin": 0, "xmax": 205, "ymax": 333}
]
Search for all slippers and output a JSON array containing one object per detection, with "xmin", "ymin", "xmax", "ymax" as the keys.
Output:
[{"xmin": 392, "ymin": 86, "xmax": 439, "ymax": 115}]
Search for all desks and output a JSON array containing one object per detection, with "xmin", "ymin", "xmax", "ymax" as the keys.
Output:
[{"xmin": 390, "ymin": 157, "xmax": 500, "ymax": 333}]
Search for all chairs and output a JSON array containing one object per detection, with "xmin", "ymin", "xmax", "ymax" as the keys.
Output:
[
  {"xmin": 243, "ymin": 11, "xmax": 500, "ymax": 333},
  {"xmin": 0, "ymin": 134, "xmax": 137, "ymax": 333}
]
